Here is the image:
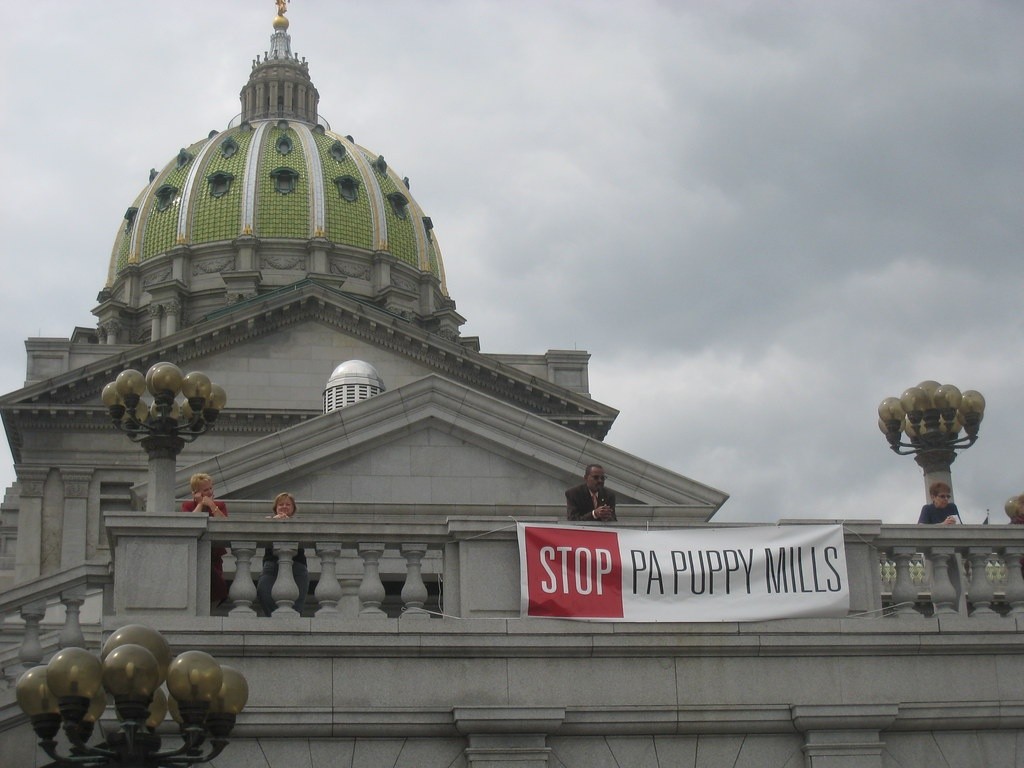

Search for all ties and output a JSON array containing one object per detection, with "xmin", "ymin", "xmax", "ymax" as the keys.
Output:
[{"xmin": 592, "ymin": 494, "xmax": 599, "ymax": 509}]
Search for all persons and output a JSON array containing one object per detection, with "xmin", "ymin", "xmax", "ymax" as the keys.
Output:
[
  {"xmin": 917, "ymin": 482, "xmax": 963, "ymax": 525},
  {"xmin": 258, "ymin": 493, "xmax": 308, "ymax": 617},
  {"xmin": 182, "ymin": 473, "xmax": 228, "ymax": 601},
  {"xmin": 565, "ymin": 464, "xmax": 616, "ymax": 521}
]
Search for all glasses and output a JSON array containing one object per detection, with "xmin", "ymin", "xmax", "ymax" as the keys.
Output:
[
  {"xmin": 937, "ymin": 495, "xmax": 951, "ymax": 498},
  {"xmin": 590, "ymin": 474, "xmax": 607, "ymax": 481}
]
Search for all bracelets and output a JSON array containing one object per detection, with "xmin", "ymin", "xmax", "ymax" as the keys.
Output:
[{"xmin": 212, "ymin": 506, "xmax": 218, "ymax": 513}]
